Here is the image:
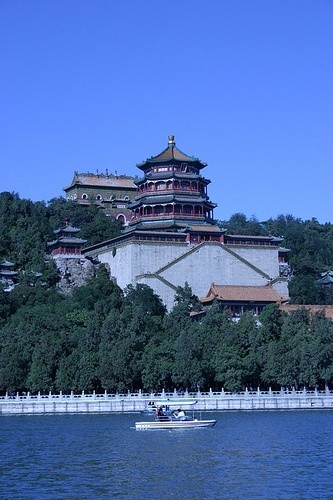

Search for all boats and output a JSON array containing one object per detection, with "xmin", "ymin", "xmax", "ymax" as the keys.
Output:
[
  {"xmin": 155, "ymin": 400, "xmax": 199, "ymax": 422},
  {"xmin": 129, "ymin": 419, "xmax": 217, "ymax": 432}
]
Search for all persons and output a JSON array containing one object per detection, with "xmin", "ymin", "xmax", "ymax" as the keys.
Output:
[
  {"xmin": 172, "ymin": 408, "xmax": 189, "ymax": 421},
  {"xmin": 151, "ymin": 402, "xmax": 157, "ymax": 411},
  {"xmin": 148, "ymin": 402, "xmax": 151, "ymax": 408}
]
